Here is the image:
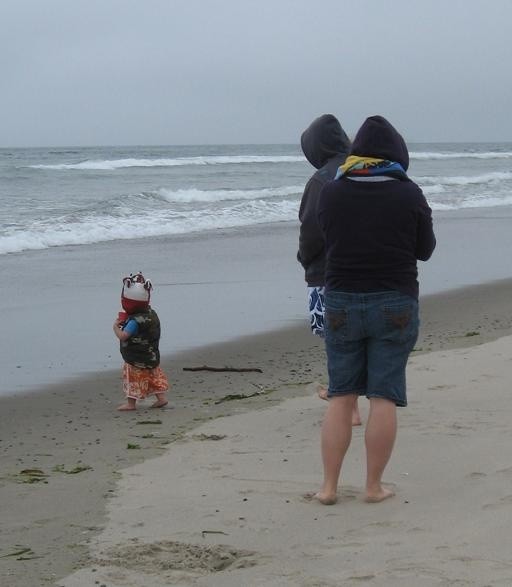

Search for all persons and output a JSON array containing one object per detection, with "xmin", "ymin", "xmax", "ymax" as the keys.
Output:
[
  {"xmin": 113, "ymin": 271, "xmax": 170, "ymax": 410},
  {"xmin": 312, "ymin": 114, "xmax": 436, "ymax": 506},
  {"xmin": 300, "ymin": 113, "xmax": 362, "ymax": 426}
]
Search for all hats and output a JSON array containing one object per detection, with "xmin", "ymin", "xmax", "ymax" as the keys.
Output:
[{"xmin": 121, "ymin": 271, "xmax": 153, "ymax": 302}]
